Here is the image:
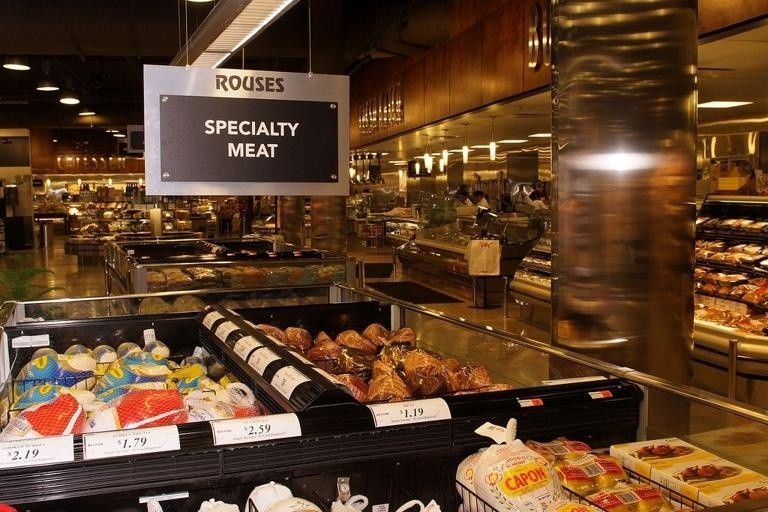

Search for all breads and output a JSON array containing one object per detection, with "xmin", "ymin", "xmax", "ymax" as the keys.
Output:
[
  {"xmin": 695, "ymin": 218, "xmax": 768, "ymax": 335},
  {"xmin": 526, "ymin": 438, "xmax": 677, "ymax": 511},
  {"xmin": 256, "ymin": 323, "xmax": 513, "ymax": 402}
]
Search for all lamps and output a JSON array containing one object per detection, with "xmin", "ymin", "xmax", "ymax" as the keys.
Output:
[
  {"xmin": 489, "ymin": 115, "xmax": 496, "ymax": 160},
  {"xmin": 462, "ymin": 122, "xmax": 470, "ymax": 164},
  {"xmin": 414, "ymin": 126, "xmax": 449, "ymax": 175}
]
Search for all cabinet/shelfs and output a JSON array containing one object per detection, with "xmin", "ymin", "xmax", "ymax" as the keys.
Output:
[{"xmin": 510, "ymin": 196, "xmax": 768, "ymax": 409}]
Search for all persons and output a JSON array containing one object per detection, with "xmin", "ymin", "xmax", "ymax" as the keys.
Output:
[
  {"xmin": 532, "ymin": 192, "xmax": 548, "ymax": 209},
  {"xmin": 457, "ymin": 184, "xmax": 489, "ymax": 208},
  {"xmin": 220, "ymin": 198, "xmax": 235, "ymax": 232}
]
